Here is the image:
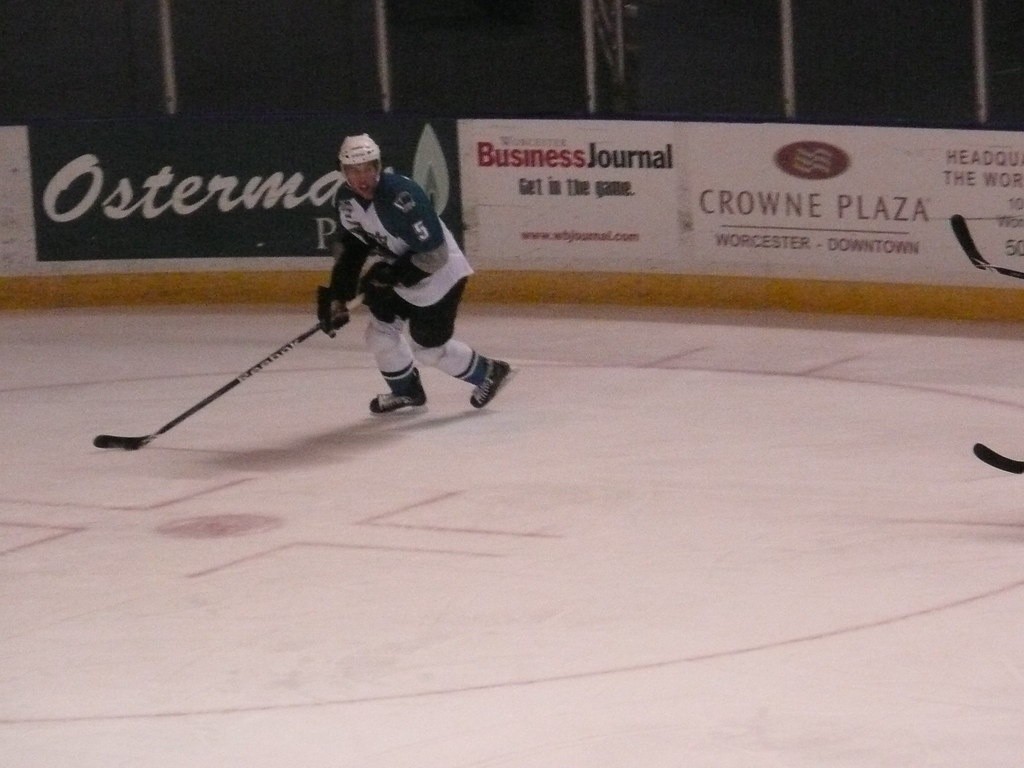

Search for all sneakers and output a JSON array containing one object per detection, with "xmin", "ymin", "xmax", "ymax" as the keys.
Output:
[
  {"xmin": 470, "ymin": 360, "xmax": 520, "ymax": 409},
  {"xmin": 370, "ymin": 367, "xmax": 429, "ymax": 416}
]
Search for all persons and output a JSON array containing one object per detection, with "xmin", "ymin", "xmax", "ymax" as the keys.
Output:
[{"xmin": 316, "ymin": 132, "xmax": 520, "ymax": 417}]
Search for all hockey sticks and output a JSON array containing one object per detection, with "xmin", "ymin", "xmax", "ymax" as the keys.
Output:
[
  {"xmin": 972, "ymin": 441, "xmax": 1024, "ymax": 475},
  {"xmin": 91, "ymin": 291, "xmax": 365, "ymax": 451},
  {"xmin": 950, "ymin": 213, "xmax": 1024, "ymax": 280}
]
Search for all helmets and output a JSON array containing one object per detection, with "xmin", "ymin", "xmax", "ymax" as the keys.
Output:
[{"xmin": 338, "ymin": 133, "xmax": 382, "ymax": 186}]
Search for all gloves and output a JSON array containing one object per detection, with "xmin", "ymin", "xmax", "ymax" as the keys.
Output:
[
  {"xmin": 370, "ymin": 266, "xmax": 399, "ymax": 288},
  {"xmin": 328, "ymin": 299, "xmax": 350, "ymax": 329}
]
